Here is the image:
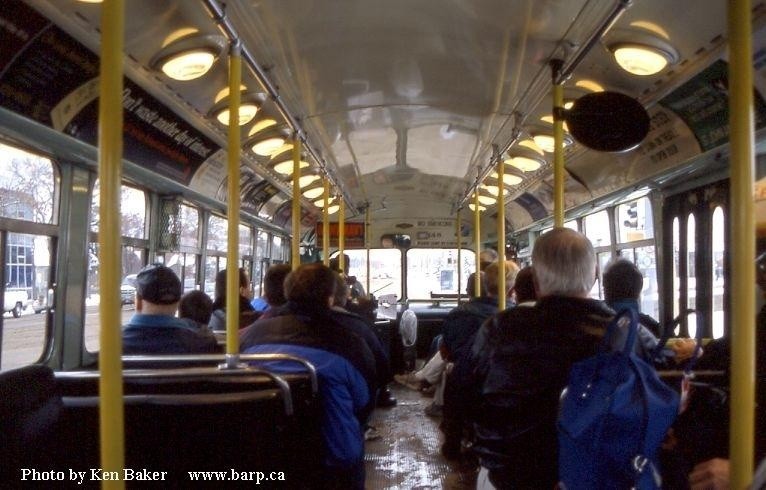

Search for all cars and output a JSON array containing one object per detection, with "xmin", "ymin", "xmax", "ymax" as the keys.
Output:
[
  {"xmin": 179, "ymin": 279, "xmax": 216, "ymax": 301},
  {"xmin": 32, "ymin": 297, "xmax": 47, "ymax": 314}
]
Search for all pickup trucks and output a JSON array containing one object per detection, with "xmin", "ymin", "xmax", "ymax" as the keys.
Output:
[{"xmin": 3, "ymin": 291, "xmax": 28, "ymax": 318}]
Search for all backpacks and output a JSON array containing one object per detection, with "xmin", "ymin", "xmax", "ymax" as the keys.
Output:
[{"xmin": 552, "ymin": 302, "xmax": 709, "ymax": 490}]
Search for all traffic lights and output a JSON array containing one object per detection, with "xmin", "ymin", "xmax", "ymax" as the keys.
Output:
[{"xmin": 621, "ymin": 194, "xmax": 645, "ymax": 229}]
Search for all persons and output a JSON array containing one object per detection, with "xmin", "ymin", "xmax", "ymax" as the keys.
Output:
[{"xmin": 121, "ymin": 177, "xmax": 766, "ymax": 490}]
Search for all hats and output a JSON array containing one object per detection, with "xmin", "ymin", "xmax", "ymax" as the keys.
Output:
[{"xmin": 133, "ymin": 259, "xmax": 183, "ymax": 306}]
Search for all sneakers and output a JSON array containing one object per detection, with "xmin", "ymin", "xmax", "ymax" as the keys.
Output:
[
  {"xmin": 376, "ymin": 397, "xmax": 398, "ymax": 408},
  {"xmin": 424, "ymin": 402, "xmax": 445, "ymax": 418},
  {"xmin": 394, "ymin": 371, "xmax": 423, "ymax": 391},
  {"xmin": 420, "ymin": 383, "xmax": 437, "ymax": 399},
  {"xmin": 364, "ymin": 424, "xmax": 380, "ymax": 443},
  {"xmin": 440, "ymin": 440, "xmax": 462, "ymax": 463},
  {"xmin": 378, "ymin": 385, "xmax": 393, "ymax": 400}
]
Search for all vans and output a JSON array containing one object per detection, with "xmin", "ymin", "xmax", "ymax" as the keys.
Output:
[{"xmin": 120, "ymin": 274, "xmax": 139, "ymax": 307}]
